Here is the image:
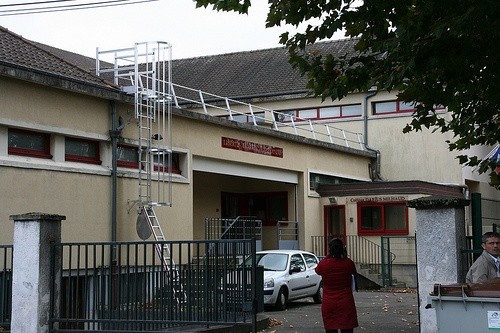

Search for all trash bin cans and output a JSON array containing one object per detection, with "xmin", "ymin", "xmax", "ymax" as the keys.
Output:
[{"xmin": 426, "ymin": 282, "xmax": 499, "ymax": 333}]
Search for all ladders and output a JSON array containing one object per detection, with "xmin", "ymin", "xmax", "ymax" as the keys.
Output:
[{"xmin": 141, "ymin": 206, "xmax": 189, "ymax": 313}]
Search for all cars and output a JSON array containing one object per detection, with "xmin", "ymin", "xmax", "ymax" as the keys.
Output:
[{"xmin": 218, "ymin": 250, "xmax": 323, "ymax": 311}]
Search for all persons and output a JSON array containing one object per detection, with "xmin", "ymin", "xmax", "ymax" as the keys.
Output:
[
  {"xmin": 466, "ymin": 231, "xmax": 500, "ymax": 289},
  {"xmin": 315, "ymin": 237, "xmax": 359, "ymax": 333}
]
